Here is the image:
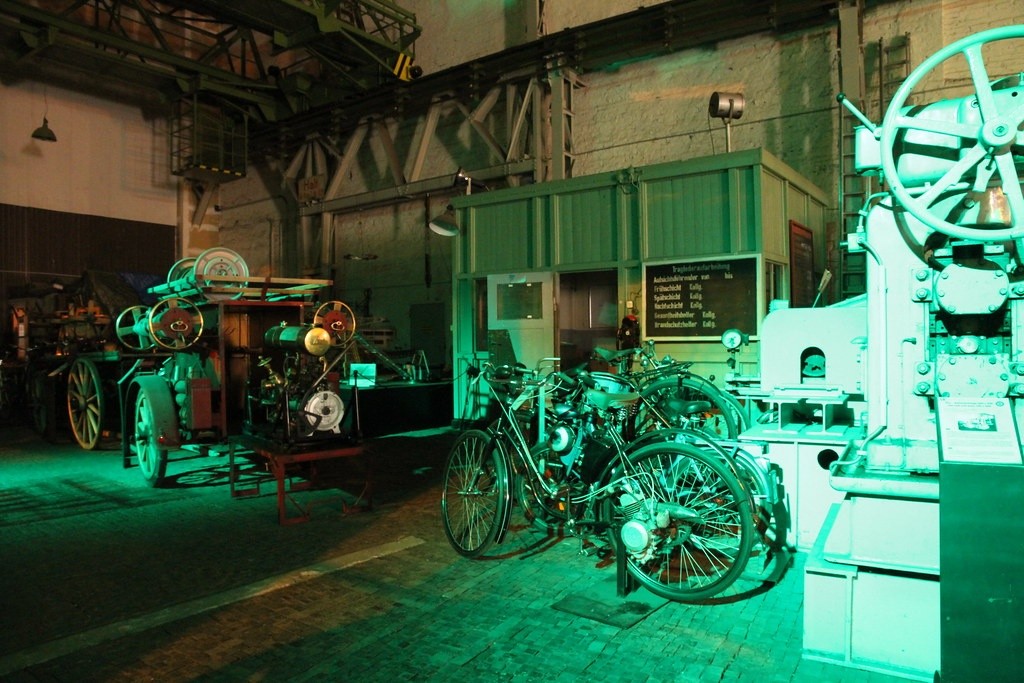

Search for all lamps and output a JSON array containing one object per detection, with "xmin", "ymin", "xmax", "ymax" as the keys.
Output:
[{"xmin": 31, "ymin": 80, "xmax": 57, "ymax": 143}]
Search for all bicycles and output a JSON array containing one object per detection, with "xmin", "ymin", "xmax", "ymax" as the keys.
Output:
[{"xmin": 440, "ymin": 343, "xmax": 786, "ymax": 604}]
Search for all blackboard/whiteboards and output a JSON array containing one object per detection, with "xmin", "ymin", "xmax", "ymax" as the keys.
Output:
[{"xmin": 642, "ymin": 254, "xmax": 762, "ymax": 341}]
